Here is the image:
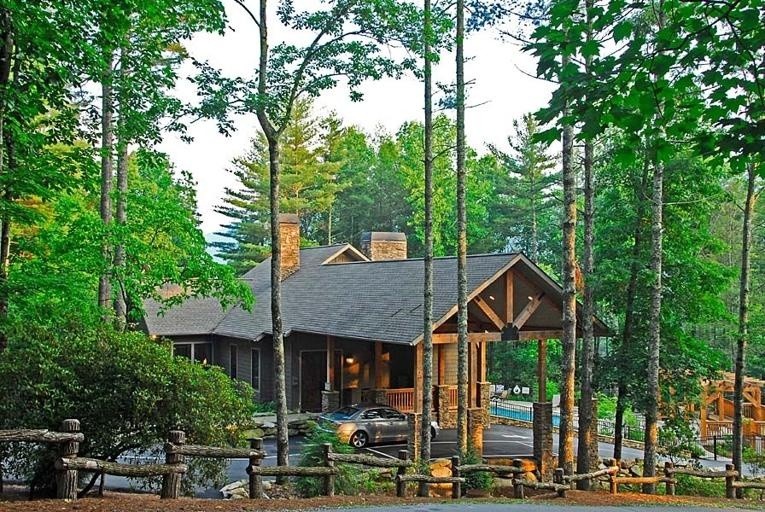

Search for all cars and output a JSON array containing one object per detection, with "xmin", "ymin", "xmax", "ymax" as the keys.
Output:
[{"xmin": 317, "ymin": 403, "xmax": 437, "ymax": 448}]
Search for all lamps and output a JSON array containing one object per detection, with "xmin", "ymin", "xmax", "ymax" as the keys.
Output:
[{"xmin": 346, "ymin": 352, "xmax": 354, "ymax": 365}]
